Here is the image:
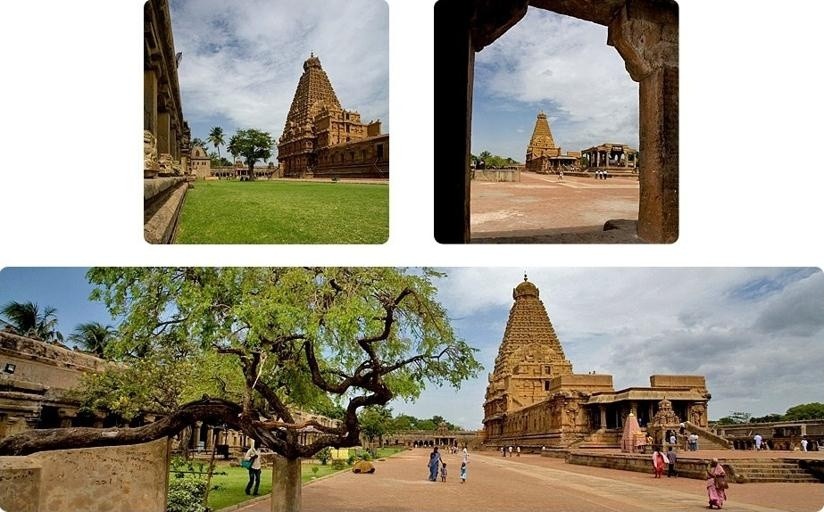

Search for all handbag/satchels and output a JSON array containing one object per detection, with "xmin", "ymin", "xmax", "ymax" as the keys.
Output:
[
  {"xmin": 241, "ymin": 461, "xmax": 251, "ymax": 468},
  {"xmin": 715, "ymin": 476, "xmax": 729, "ymax": 489}
]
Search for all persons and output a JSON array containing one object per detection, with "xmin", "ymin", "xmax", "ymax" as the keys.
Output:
[
  {"xmin": 500, "ymin": 443, "xmax": 520, "ymax": 458},
  {"xmin": 244, "ymin": 439, "xmax": 262, "ymax": 496},
  {"xmin": 706, "ymin": 458, "xmax": 726, "ymax": 509},
  {"xmin": 541, "ymin": 444, "xmax": 546, "ymax": 452},
  {"xmin": 595, "ymin": 169, "xmax": 608, "ymax": 180},
  {"xmin": 621, "ymin": 410, "xmax": 701, "ymax": 478},
  {"xmin": 734, "ymin": 433, "xmax": 813, "ymax": 452},
  {"xmin": 429, "ymin": 446, "xmax": 469, "ymax": 483}
]
